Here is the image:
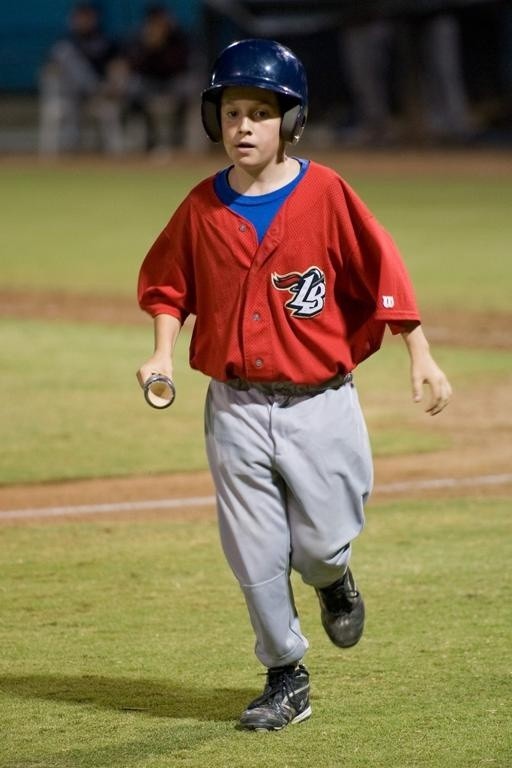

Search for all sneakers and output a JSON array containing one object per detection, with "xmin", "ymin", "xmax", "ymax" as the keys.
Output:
[
  {"xmin": 240, "ymin": 664, "xmax": 314, "ymax": 731},
  {"xmin": 314, "ymin": 566, "xmax": 366, "ymax": 649}
]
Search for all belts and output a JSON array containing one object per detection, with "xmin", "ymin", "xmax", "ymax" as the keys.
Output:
[{"xmin": 223, "ymin": 373, "xmax": 354, "ymax": 396}]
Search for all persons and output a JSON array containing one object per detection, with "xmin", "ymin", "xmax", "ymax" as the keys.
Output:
[{"xmin": 136, "ymin": 37, "xmax": 456, "ymax": 729}]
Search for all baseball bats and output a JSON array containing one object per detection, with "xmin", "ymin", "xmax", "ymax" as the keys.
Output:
[{"xmin": 143, "ymin": 373, "xmax": 181, "ymax": 408}]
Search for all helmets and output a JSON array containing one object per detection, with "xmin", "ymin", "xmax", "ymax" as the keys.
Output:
[{"xmin": 201, "ymin": 36, "xmax": 308, "ymax": 145}]
